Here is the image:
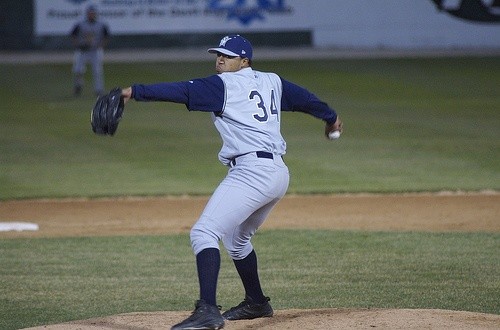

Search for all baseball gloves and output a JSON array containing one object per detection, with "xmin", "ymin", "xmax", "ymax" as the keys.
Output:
[{"xmin": 89, "ymin": 86, "xmax": 124, "ymax": 136}]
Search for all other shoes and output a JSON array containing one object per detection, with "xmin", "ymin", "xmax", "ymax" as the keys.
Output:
[
  {"xmin": 170, "ymin": 302, "xmax": 225, "ymax": 330},
  {"xmin": 221, "ymin": 296, "xmax": 273, "ymax": 319}
]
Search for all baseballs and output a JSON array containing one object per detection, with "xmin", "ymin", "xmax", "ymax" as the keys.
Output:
[{"xmin": 328, "ymin": 129, "xmax": 342, "ymax": 140}]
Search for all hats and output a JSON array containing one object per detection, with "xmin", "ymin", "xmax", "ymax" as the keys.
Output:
[{"xmin": 207, "ymin": 34, "xmax": 253, "ymax": 64}]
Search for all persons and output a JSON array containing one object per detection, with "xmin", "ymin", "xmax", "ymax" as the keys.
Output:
[
  {"xmin": 69, "ymin": 6, "xmax": 111, "ymax": 99},
  {"xmin": 118, "ymin": 36, "xmax": 344, "ymax": 330}
]
